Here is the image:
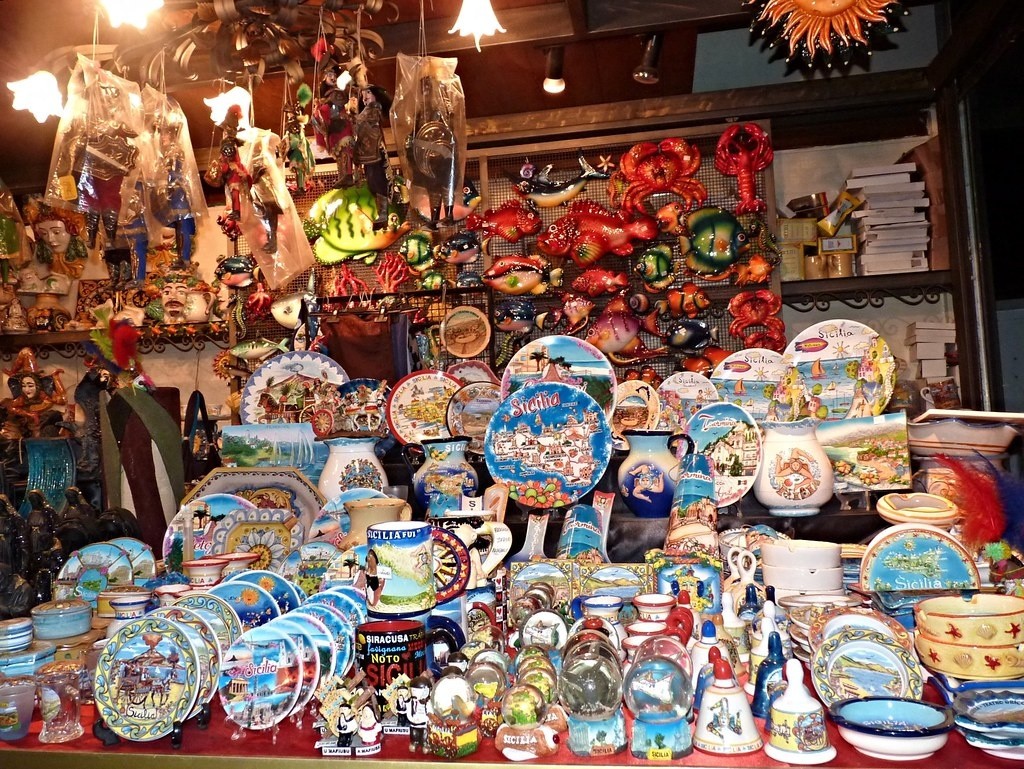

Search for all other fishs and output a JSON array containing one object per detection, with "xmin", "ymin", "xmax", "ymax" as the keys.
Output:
[{"xmin": 213, "ymin": 122, "xmax": 788, "ymax": 387}]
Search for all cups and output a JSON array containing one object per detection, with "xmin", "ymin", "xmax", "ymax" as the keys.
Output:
[
  {"xmin": 0, "ymin": 680, "xmax": 35, "ymax": 740},
  {"xmin": 365, "ymin": 521, "xmax": 471, "ymax": 619},
  {"xmin": 36, "ymin": 673, "xmax": 85, "ymax": 743},
  {"xmin": 427, "ymin": 509, "xmax": 513, "ymax": 590},
  {"xmin": 359, "ymin": 591, "xmax": 502, "ymax": 691}
]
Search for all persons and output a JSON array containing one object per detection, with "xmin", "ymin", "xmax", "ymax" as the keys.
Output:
[
  {"xmin": 356, "ymin": 706, "xmax": 382, "ymax": 745},
  {"xmin": 353, "ymin": 80, "xmax": 394, "ymax": 197},
  {"xmin": 411, "ymin": 75, "xmax": 458, "ymax": 229},
  {"xmin": 355, "ymin": 83, "xmax": 397, "ymax": 221},
  {"xmin": 393, "ymin": 687, "xmax": 410, "ymax": 725},
  {"xmin": 5, "ymin": 373, "xmax": 54, "ymax": 436},
  {"xmin": 244, "ymin": 137, "xmax": 284, "ymax": 255},
  {"xmin": 204, "ymin": 105, "xmax": 250, "ymax": 224},
  {"xmin": 335, "ymin": 704, "xmax": 357, "ymax": 747},
  {"xmin": 404, "ymin": 677, "xmax": 433, "ymax": 755},
  {"xmin": 313, "ymin": 72, "xmax": 354, "ymax": 186}
]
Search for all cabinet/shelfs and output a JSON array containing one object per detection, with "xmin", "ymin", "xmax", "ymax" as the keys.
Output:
[{"xmin": 1, "ymin": 57, "xmax": 1008, "ymax": 414}]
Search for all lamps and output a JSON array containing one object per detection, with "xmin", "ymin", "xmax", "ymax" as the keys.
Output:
[
  {"xmin": 543, "ymin": 48, "xmax": 566, "ymax": 92},
  {"xmin": 633, "ymin": 33, "xmax": 662, "ymax": 86}
]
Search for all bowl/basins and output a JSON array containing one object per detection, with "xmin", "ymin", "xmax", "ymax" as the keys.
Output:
[{"xmin": 828, "ymin": 696, "xmax": 955, "ymax": 761}]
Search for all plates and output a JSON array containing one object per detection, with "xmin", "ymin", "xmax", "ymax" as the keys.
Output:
[
  {"xmin": 675, "ymin": 401, "xmax": 764, "ymax": 508},
  {"xmin": 54, "ymin": 537, "xmax": 156, "ymax": 611},
  {"xmin": 174, "ymin": 350, "xmax": 502, "ymax": 547},
  {"xmin": 613, "ymin": 319, "xmax": 897, "ymax": 448},
  {"xmin": 950, "ymin": 687, "xmax": 1024, "ymax": 760},
  {"xmin": 860, "ymin": 524, "xmax": 984, "ymax": 628},
  {"xmin": 162, "ymin": 493, "xmax": 258, "ymax": 580},
  {"xmin": 219, "ymin": 587, "xmax": 367, "ymax": 730},
  {"xmin": 308, "ymin": 487, "xmax": 390, "ymax": 551},
  {"xmin": 809, "ymin": 605, "xmax": 923, "ymax": 708},
  {"xmin": 94, "ymin": 541, "xmax": 342, "ymax": 741},
  {"xmin": 483, "ymin": 334, "xmax": 617, "ymax": 508}
]
